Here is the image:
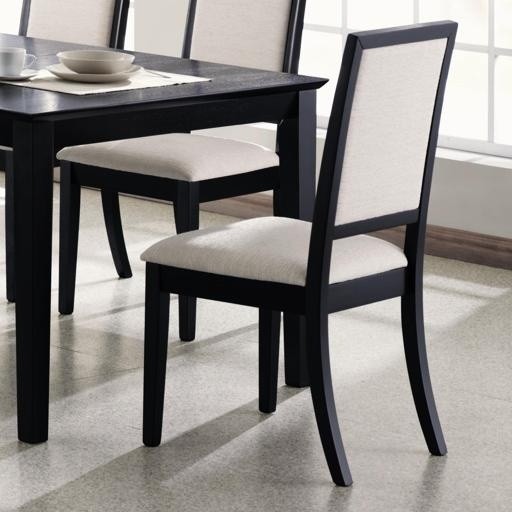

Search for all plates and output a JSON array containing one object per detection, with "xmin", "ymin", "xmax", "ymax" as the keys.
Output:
[
  {"xmin": 47, "ymin": 63, "xmax": 144, "ymax": 85},
  {"xmin": 0, "ymin": 67, "xmax": 37, "ymax": 83}
]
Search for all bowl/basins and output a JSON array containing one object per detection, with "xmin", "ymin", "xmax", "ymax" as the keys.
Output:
[{"xmin": 54, "ymin": 48, "xmax": 136, "ymax": 74}]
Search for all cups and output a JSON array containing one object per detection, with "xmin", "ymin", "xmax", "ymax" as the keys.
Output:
[{"xmin": 0, "ymin": 47, "xmax": 37, "ymax": 76}]
